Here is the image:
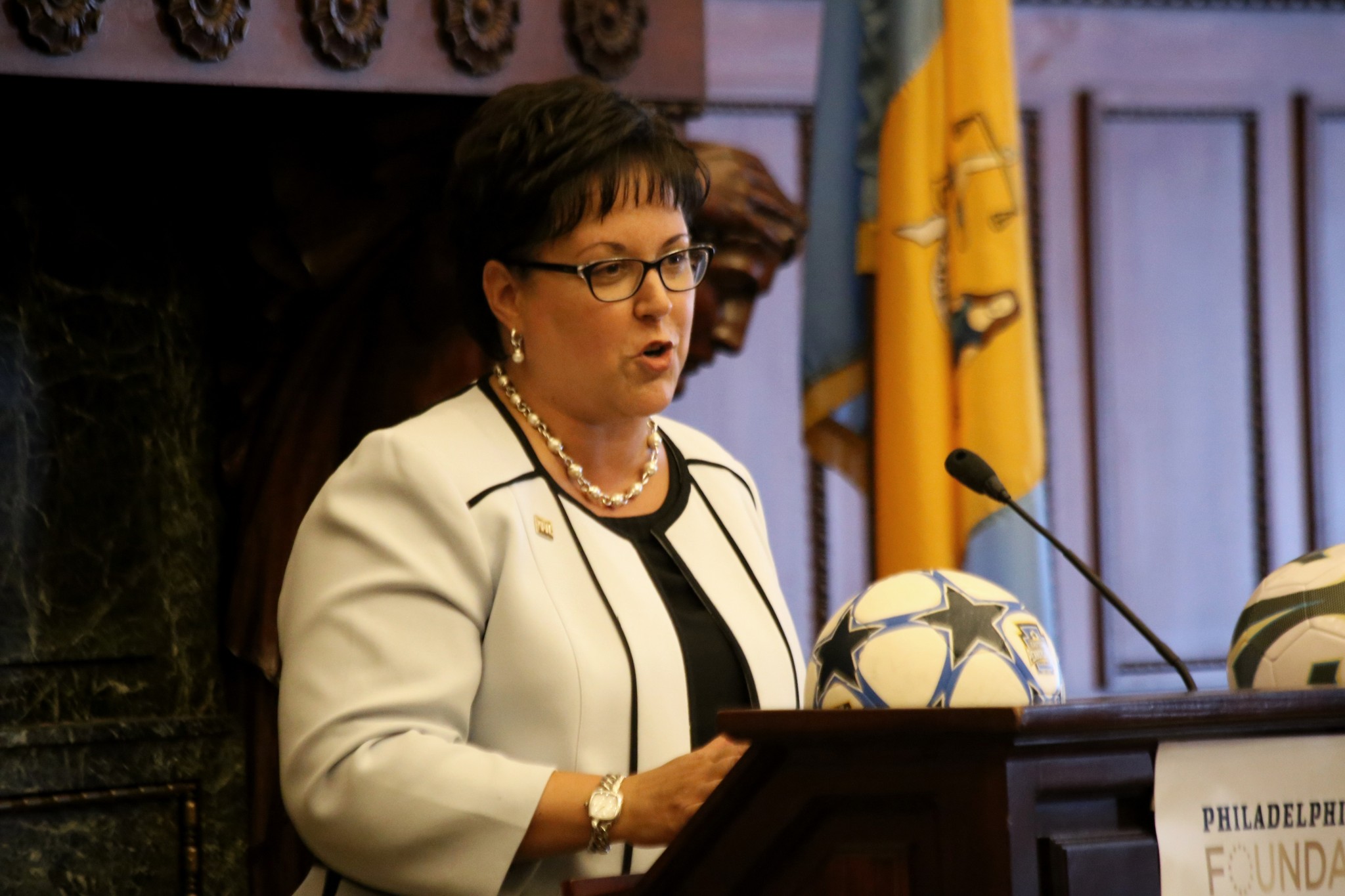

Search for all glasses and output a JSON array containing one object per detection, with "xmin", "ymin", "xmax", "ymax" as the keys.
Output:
[{"xmin": 511, "ymin": 243, "xmax": 715, "ymax": 303}]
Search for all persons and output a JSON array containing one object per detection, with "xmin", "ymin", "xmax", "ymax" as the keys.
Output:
[
  {"xmin": 670, "ymin": 135, "xmax": 805, "ymax": 397},
  {"xmin": 275, "ymin": 82, "xmax": 813, "ymax": 896}
]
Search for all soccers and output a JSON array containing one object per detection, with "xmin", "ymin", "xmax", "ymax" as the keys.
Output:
[
  {"xmin": 805, "ymin": 569, "xmax": 1065, "ymax": 709},
  {"xmin": 1227, "ymin": 545, "xmax": 1345, "ymax": 690}
]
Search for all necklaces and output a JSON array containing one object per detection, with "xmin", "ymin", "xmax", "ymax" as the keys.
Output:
[{"xmin": 487, "ymin": 360, "xmax": 661, "ymax": 507}]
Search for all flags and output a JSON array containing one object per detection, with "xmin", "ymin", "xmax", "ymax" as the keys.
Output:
[{"xmin": 799, "ymin": 1, "xmax": 1044, "ymax": 633}]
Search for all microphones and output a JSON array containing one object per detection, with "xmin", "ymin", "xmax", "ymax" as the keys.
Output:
[{"xmin": 945, "ymin": 448, "xmax": 1199, "ymax": 692}]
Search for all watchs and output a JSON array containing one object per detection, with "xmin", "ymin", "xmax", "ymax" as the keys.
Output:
[{"xmin": 583, "ymin": 771, "xmax": 625, "ymax": 852}]
